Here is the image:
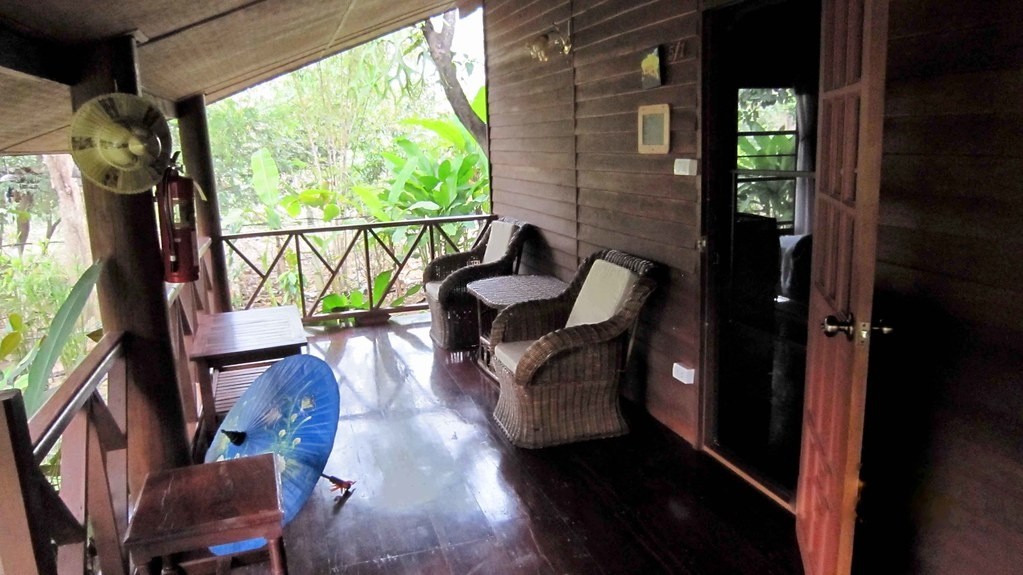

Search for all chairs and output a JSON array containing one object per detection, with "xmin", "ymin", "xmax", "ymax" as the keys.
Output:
[
  {"xmin": 423, "ymin": 216, "xmax": 528, "ymax": 350},
  {"xmin": 487, "ymin": 248, "xmax": 656, "ymax": 448}
]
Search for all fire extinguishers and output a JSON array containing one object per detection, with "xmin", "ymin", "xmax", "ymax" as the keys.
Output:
[{"xmin": 156, "ymin": 150, "xmax": 201, "ymax": 284}]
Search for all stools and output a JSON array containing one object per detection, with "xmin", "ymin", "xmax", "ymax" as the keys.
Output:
[{"xmin": 122, "ymin": 452, "xmax": 289, "ymax": 575}]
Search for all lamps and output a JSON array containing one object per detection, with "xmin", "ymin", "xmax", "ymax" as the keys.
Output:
[{"xmin": 525, "ymin": 29, "xmax": 573, "ymax": 63}]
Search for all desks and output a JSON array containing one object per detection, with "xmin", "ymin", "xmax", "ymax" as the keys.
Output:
[
  {"xmin": 467, "ymin": 272, "xmax": 569, "ymax": 383},
  {"xmin": 188, "ymin": 303, "xmax": 308, "ymax": 447}
]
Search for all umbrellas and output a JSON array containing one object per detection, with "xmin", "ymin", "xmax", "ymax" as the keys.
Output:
[{"xmin": 203, "ymin": 354, "xmax": 351, "ymax": 556}]
